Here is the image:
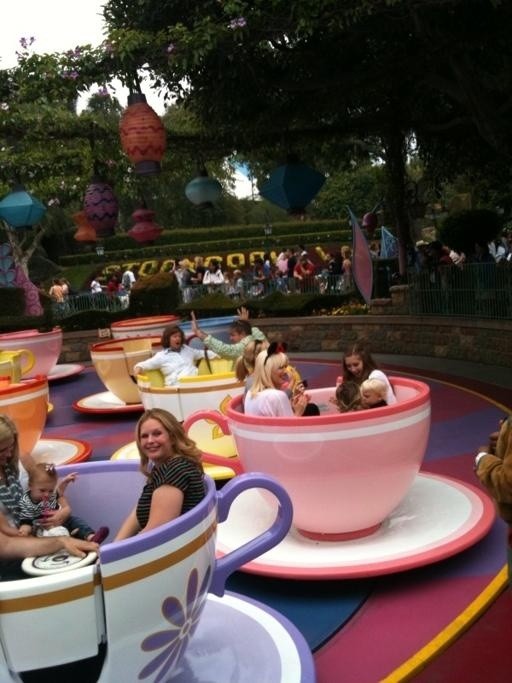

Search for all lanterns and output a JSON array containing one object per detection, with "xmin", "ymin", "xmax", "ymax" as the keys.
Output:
[
  {"xmin": 185, "ymin": 154, "xmax": 221, "ymax": 212},
  {"xmin": 85, "ymin": 173, "xmax": 119, "ymax": 239},
  {"xmin": 118, "ymin": 67, "xmax": 166, "ymax": 177},
  {"xmin": 0, "ymin": 184, "xmax": 47, "ymax": 233},
  {"xmin": 129, "ymin": 196, "xmax": 163, "ymax": 245},
  {"xmin": 258, "ymin": 140, "xmax": 326, "ymax": 214},
  {"xmin": 71, "ymin": 207, "xmax": 96, "ymax": 243}
]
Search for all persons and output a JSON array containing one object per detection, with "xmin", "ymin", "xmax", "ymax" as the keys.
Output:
[
  {"xmin": 243, "ymin": 341, "xmax": 308, "ymax": 417},
  {"xmin": 133, "ymin": 326, "xmax": 219, "ymax": 387},
  {"xmin": 362, "ymin": 378, "xmax": 387, "ymax": 408},
  {"xmin": 112, "ymin": 407, "xmax": 204, "ymax": 545},
  {"xmin": 410, "ymin": 228, "xmax": 512, "ymax": 287},
  {"xmin": 235, "ymin": 340, "xmax": 321, "ymax": 416},
  {"xmin": 190, "ymin": 306, "xmax": 267, "ymax": 370},
  {"xmin": 343, "ymin": 342, "xmax": 398, "ymax": 405},
  {"xmin": 335, "ymin": 381, "xmax": 369, "ymax": 413},
  {"xmin": 18, "ymin": 462, "xmax": 109, "ymax": 545},
  {"xmin": 33, "ymin": 275, "xmax": 71, "ymax": 316},
  {"xmin": 476, "ymin": 415, "xmax": 512, "ymax": 583},
  {"xmin": 1, "ymin": 414, "xmax": 102, "ymax": 580},
  {"xmin": 93, "ymin": 231, "xmax": 381, "ymax": 307}
]
